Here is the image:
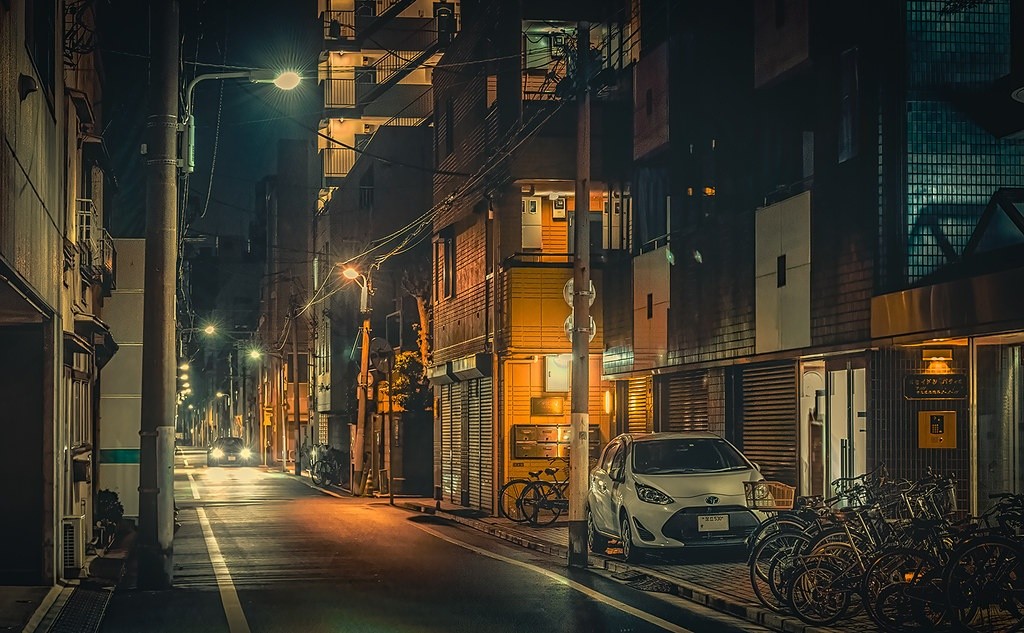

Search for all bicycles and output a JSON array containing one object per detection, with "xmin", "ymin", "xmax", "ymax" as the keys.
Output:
[
  {"xmin": 311, "ymin": 443, "xmax": 344, "ymax": 486},
  {"xmin": 499, "ymin": 455, "xmax": 600, "ymax": 525},
  {"xmin": 739, "ymin": 459, "xmax": 1024, "ymax": 633}
]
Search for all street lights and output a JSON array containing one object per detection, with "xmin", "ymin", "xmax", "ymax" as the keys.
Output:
[
  {"xmin": 251, "ymin": 351, "xmax": 265, "ymax": 467},
  {"xmin": 343, "ymin": 266, "xmax": 375, "ymax": 494},
  {"xmin": 137, "ymin": 0, "xmax": 301, "ymax": 592}
]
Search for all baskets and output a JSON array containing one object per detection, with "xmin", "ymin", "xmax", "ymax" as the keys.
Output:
[{"xmin": 742, "ymin": 481, "xmax": 798, "ymax": 512}]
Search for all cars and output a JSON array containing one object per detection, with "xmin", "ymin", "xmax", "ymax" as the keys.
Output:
[
  {"xmin": 587, "ymin": 430, "xmax": 780, "ymax": 563},
  {"xmin": 207, "ymin": 436, "xmax": 250, "ymax": 467}
]
